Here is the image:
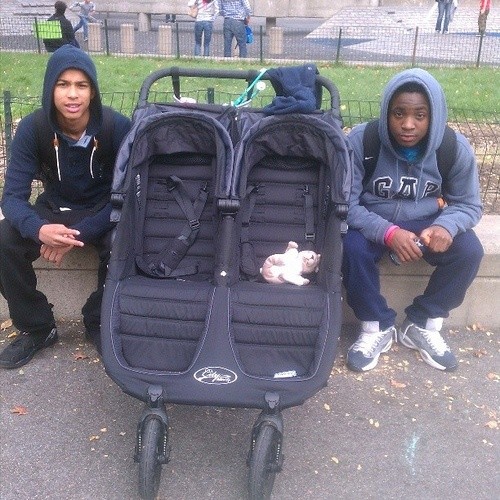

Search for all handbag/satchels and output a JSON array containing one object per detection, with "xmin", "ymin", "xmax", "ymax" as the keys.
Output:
[
  {"xmin": 189, "ymin": 0, "xmax": 198, "ymax": 18},
  {"xmin": 244, "ymin": 26, "xmax": 253, "ymax": 43}
]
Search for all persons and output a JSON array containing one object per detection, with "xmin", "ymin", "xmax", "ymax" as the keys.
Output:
[
  {"xmin": 434, "ymin": 0, "xmax": 457, "ymax": 35},
  {"xmin": 42, "ymin": 1, "xmax": 80, "ymax": 52},
  {"xmin": 342, "ymin": 68, "xmax": 484, "ymax": 372},
  {"xmin": 188, "ymin": 0, "xmax": 219, "ymax": 56},
  {"xmin": 475, "ymin": 0, "xmax": 490, "ymax": 37},
  {"xmin": 0, "ymin": 43, "xmax": 133, "ymax": 369},
  {"xmin": 218, "ymin": 0, "xmax": 251, "ymax": 58},
  {"xmin": 69, "ymin": 0, "xmax": 96, "ymax": 42}
]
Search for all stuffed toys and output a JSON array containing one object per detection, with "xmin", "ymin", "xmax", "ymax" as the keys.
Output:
[{"xmin": 260, "ymin": 241, "xmax": 322, "ymax": 286}]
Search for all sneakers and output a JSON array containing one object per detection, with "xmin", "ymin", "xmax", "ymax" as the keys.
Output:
[
  {"xmin": 85, "ymin": 323, "xmax": 103, "ymax": 357},
  {"xmin": 0, "ymin": 303, "xmax": 58, "ymax": 368},
  {"xmin": 399, "ymin": 317, "xmax": 459, "ymax": 371},
  {"xmin": 346, "ymin": 321, "xmax": 397, "ymax": 372}
]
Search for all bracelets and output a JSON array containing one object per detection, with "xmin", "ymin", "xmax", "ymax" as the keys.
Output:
[{"xmin": 384, "ymin": 225, "xmax": 399, "ymax": 252}]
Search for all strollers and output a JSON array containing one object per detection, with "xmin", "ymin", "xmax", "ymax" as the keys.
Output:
[{"xmin": 101, "ymin": 67, "xmax": 355, "ymax": 500}]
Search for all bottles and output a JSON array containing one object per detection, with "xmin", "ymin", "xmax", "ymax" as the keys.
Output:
[{"xmin": 389, "ymin": 238, "xmax": 426, "ymax": 266}]
